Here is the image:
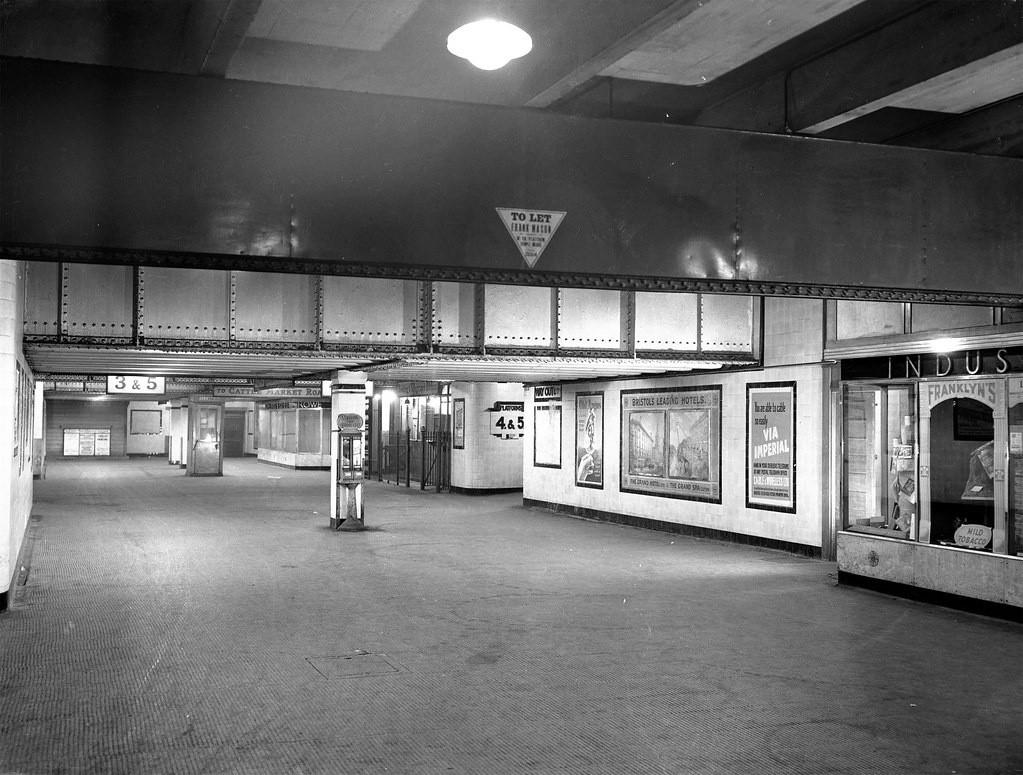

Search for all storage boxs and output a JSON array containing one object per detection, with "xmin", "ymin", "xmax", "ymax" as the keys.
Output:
[
  {"xmin": 870, "ymin": 516, "xmax": 886, "ymax": 528},
  {"xmin": 895, "ymin": 514, "xmax": 911, "ymax": 532},
  {"xmin": 856, "ymin": 518, "xmax": 870, "ymax": 526},
  {"xmin": 889, "ymin": 518, "xmax": 896, "ymax": 529}
]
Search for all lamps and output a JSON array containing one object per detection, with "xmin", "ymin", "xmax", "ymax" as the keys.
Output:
[
  {"xmin": 404, "ymin": 397, "xmax": 410, "ymax": 405},
  {"xmin": 445, "ymin": 0, "xmax": 533, "ymax": 70}
]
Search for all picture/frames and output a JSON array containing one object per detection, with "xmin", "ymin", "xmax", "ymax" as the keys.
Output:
[
  {"xmin": 745, "ymin": 380, "xmax": 798, "ymax": 516},
  {"xmin": 453, "ymin": 398, "xmax": 465, "ymax": 450},
  {"xmin": 575, "ymin": 391, "xmax": 605, "ymax": 491},
  {"xmin": 619, "ymin": 383, "xmax": 723, "ymax": 506},
  {"xmin": 534, "ymin": 406, "xmax": 562, "ymax": 470}
]
